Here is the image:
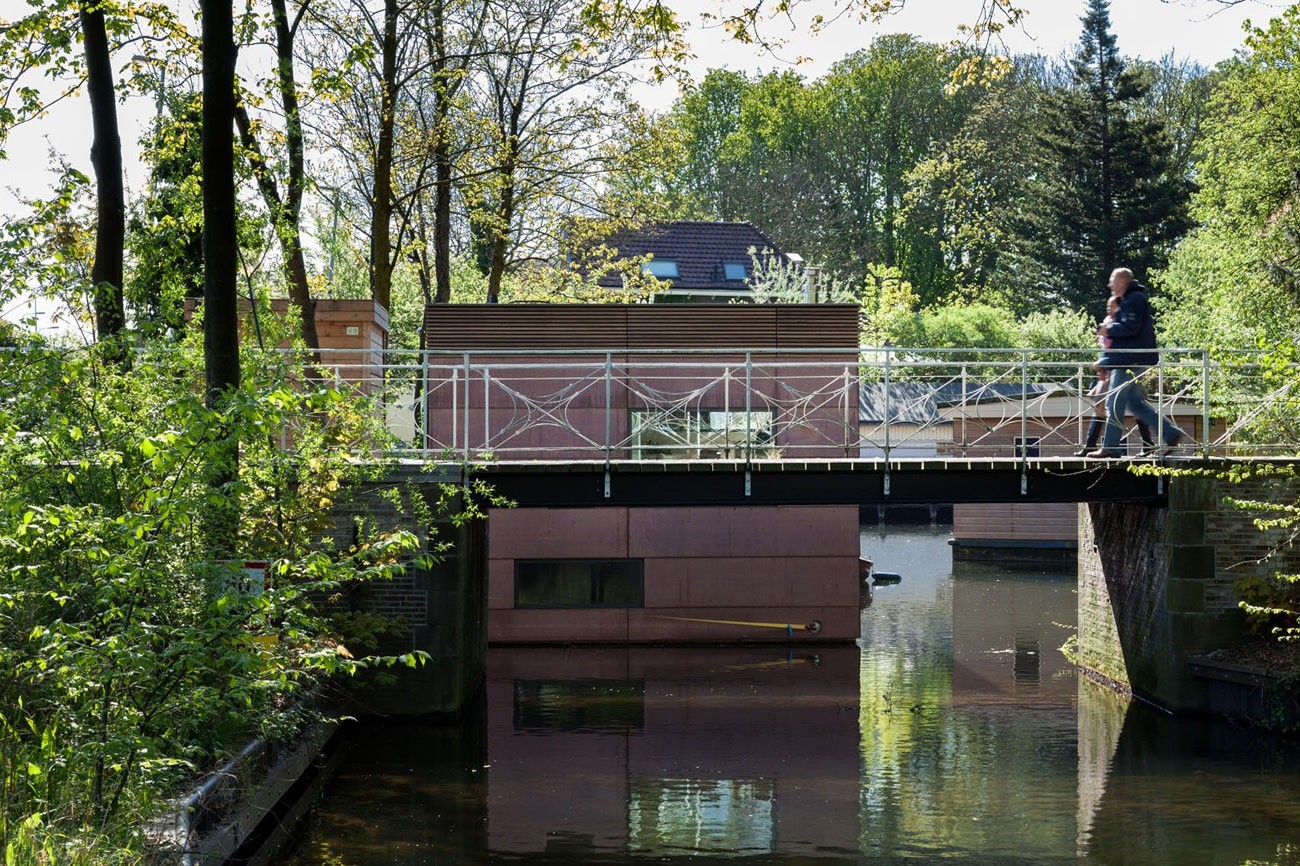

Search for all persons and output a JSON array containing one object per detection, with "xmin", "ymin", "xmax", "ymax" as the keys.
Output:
[{"xmin": 1074, "ymin": 267, "xmax": 1184, "ymax": 459}]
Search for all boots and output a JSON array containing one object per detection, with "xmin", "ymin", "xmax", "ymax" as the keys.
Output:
[
  {"xmin": 1134, "ymin": 418, "xmax": 1156, "ymax": 456},
  {"xmin": 1074, "ymin": 416, "xmax": 1106, "ymax": 456}
]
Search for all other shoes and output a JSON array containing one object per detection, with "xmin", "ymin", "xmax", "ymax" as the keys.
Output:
[
  {"xmin": 1164, "ymin": 437, "xmax": 1181, "ymax": 457},
  {"xmin": 1085, "ymin": 447, "xmax": 1122, "ymax": 458}
]
